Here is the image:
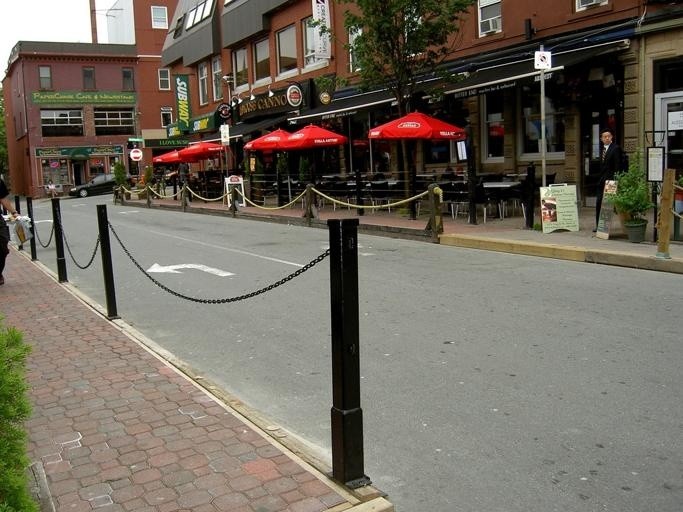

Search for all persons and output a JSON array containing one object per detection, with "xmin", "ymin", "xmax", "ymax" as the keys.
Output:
[
  {"xmin": 0, "ymin": 178, "xmax": 18, "ymax": 285},
  {"xmin": 593, "ymin": 129, "xmax": 626, "ymax": 232},
  {"xmin": 47, "ymin": 180, "xmax": 58, "ymax": 198}
]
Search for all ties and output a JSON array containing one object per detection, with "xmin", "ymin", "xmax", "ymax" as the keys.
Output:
[{"xmin": 601, "ymin": 147, "xmax": 606, "ymax": 164}]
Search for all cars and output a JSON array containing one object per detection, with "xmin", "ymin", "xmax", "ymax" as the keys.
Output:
[
  {"xmin": 68, "ymin": 172, "xmax": 135, "ymax": 198},
  {"xmin": 164, "ymin": 168, "xmax": 184, "ymax": 186}
]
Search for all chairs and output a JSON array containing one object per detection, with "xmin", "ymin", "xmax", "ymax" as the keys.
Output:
[{"xmin": 191, "ymin": 171, "xmax": 558, "ymax": 221}]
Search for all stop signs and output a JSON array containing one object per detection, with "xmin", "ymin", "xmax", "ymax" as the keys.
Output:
[{"xmin": 129, "ymin": 148, "xmax": 142, "ymax": 161}]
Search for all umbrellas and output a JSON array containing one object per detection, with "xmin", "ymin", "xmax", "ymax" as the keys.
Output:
[
  {"xmin": 243, "ymin": 123, "xmax": 349, "ymax": 150},
  {"xmin": 367, "ymin": 109, "xmax": 466, "ymax": 140},
  {"xmin": 152, "ymin": 140, "xmax": 225, "ymax": 171}
]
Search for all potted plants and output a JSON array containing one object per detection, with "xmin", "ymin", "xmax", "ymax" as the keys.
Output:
[
  {"xmin": 604, "ymin": 146, "xmax": 660, "ymax": 243},
  {"xmin": 114, "ymin": 161, "xmax": 160, "ymax": 200}
]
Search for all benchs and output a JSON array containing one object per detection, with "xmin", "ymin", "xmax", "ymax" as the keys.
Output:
[{"xmin": 45, "ymin": 184, "xmax": 64, "ymax": 198}]
